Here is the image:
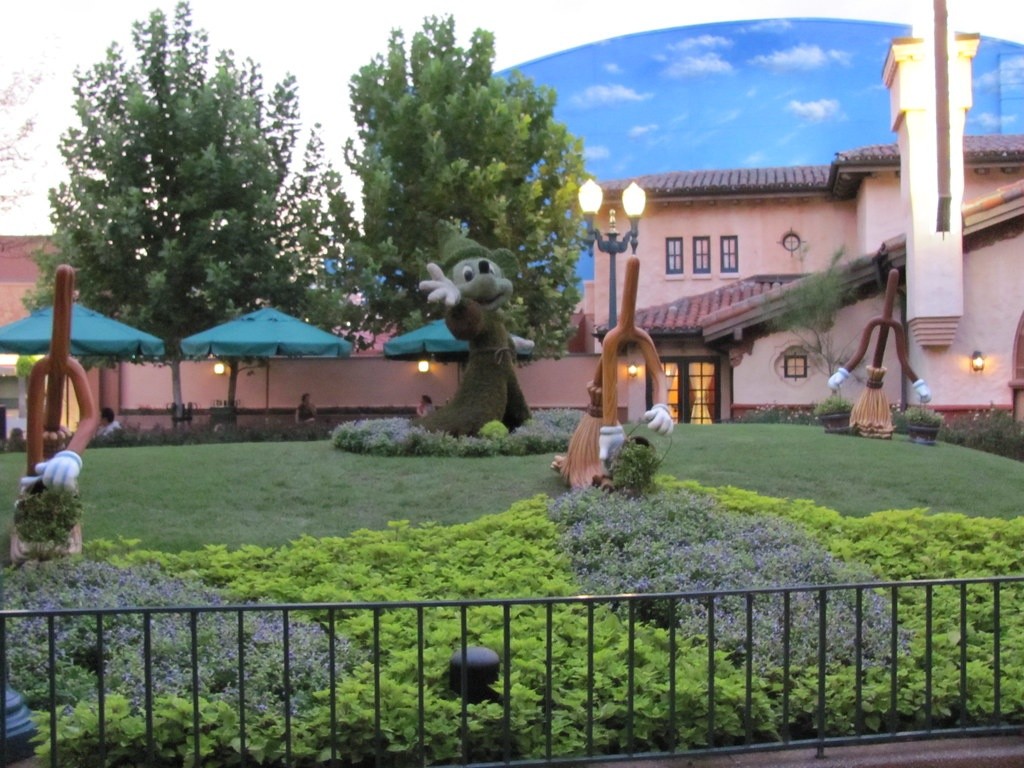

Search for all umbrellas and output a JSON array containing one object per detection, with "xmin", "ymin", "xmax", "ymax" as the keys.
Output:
[
  {"xmin": 180, "ymin": 305, "xmax": 354, "ymax": 360},
  {"xmin": 0, "ymin": 296, "xmax": 166, "ymax": 357},
  {"xmin": 383, "ymin": 316, "xmax": 535, "ymax": 363}
]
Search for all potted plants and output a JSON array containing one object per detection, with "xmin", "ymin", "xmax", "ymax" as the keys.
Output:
[
  {"xmin": 904, "ymin": 406, "xmax": 946, "ymax": 445},
  {"xmin": 13, "ymin": 480, "xmax": 83, "ymax": 549},
  {"xmin": 605, "ymin": 436, "xmax": 662, "ymax": 488},
  {"xmin": 813, "ymin": 396, "xmax": 855, "ymax": 434}
]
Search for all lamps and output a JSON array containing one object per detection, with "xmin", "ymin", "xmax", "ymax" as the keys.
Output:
[
  {"xmin": 577, "ymin": 179, "xmax": 603, "ymax": 211},
  {"xmin": 622, "ymin": 180, "xmax": 646, "ymax": 216},
  {"xmin": 213, "ymin": 362, "xmax": 225, "ymax": 375},
  {"xmin": 628, "ymin": 361, "xmax": 638, "ymax": 377},
  {"xmin": 418, "ymin": 359, "xmax": 430, "ymax": 373},
  {"xmin": 971, "ymin": 350, "xmax": 985, "ymax": 371}
]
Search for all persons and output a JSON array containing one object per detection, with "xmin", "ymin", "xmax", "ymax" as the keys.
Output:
[
  {"xmin": 4, "ymin": 427, "xmax": 26, "ymax": 452},
  {"xmin": 417, "ymin": 395, "xmax": 435, "ymax": 418},
  {"xmin": 93, "ymin": 407, "xmax": 122, "ymax": 440},
  {"xmin": 295, "ymin": 392, "xmax": 317, "ymax": 424}
]
